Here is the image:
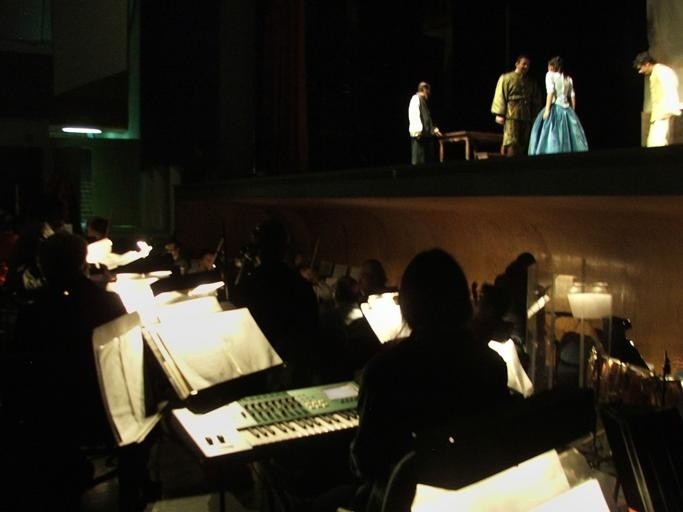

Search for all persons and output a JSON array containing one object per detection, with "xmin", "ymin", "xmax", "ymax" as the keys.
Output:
[
  {"xmin": 406, "ymin": 82, "xmax": 444, "ymax": 165},
  {"xmin": 526, "ymin": 56, "xmax": 591, "ymax": 157},
  {"xmin": 486, "ymin": 54, "xmax": 533, "ymax": 156},
  {"xmin": 634, "ymin": 51, "xmax": 682, "ymax": 150},
  {"xmin": 308, "ymin": 243, "xmax": 508, "ymax": 512},
  {"xmin": 470, "ymin": 250, "xmax": 548, "ymax": 368}
]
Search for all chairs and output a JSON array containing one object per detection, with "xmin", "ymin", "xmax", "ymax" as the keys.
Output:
[{"xmin": 366, "ymin": 384, "xmax": 596, "ymax": 510}]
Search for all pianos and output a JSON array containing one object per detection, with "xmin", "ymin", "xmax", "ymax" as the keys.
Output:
[{"xmin": 171, "ymin": 381, "xmax": 360, "ymax": 464}]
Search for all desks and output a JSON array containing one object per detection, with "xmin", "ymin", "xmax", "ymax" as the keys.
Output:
[{"xmin": 437, "ymin": 130, "xmax": 503, "ymax": 164}]
